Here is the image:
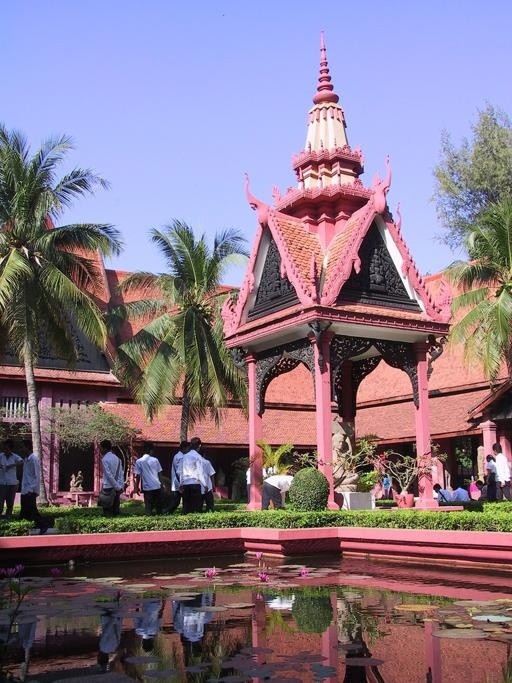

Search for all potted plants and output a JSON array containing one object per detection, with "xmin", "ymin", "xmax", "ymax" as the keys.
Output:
[{"xmin": 338, "ymin": 438, "xmax": 449, "ymax": 512}]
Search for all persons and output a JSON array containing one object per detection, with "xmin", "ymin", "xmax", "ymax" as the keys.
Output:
[
  {"xmin": 99, "ymin": 440, "xmax": 125, "ymax": 518},
  {"xmin": 433, "ymin": 484, "xmax": 452, "ymax": 502},
  {"xmin": 489, "ymin": 443, "xmax": 512, "ymax": 501},
  {"xmin": 450, "ymin": 480, "xmax": 470, "ymax": 501},
  {"xmin": 384, "ymin": 474, "xmax": 391, "ymax": 499},
  {"xmin": 0, "ymin": 439, "xmax": 27, "ymax": 518},
  {"xmin": 97, "ymin": 592, "xmax": 214, "ymax": 666},
  {"xmin": 261, "ymin": 474, "xmax": 294, "ymax": 510},
  {"xmin": 18, "ymin": 439, "xmax": 49, "ymax": 535},
  {"xmin": 163, "ymin": 437, "xmax": 215, "ymax": 514},
  {"xmin": 246, "ymin": 464, "xmax": 280, "ymax": 502},
  {"xmin": 476, "ymin": 454, "xmax": 495, "ymax": 500},
  {"xmin": 135, "ymin": 442, "xmax": 166, "ymax": 516}
]
